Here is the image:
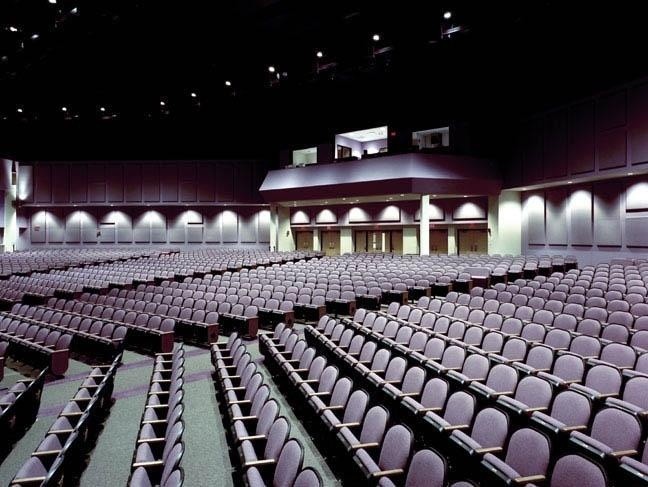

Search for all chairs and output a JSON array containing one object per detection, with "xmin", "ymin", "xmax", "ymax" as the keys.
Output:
[{"xmin": 0, "ymin": 242, "xmax": 646, "ymax": 485}]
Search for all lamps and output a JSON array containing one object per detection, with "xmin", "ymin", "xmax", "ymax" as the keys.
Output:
[
  {"xmin": 189, "ymin": 88, "xmax": 210, "ymax": 113},
  {"xmin": 365, "ymin": 30, "xmax": 401, "ymax": 65},
  {"xmin": 434, "ymin": 9, "xmax": 464, "ymax": 42},
  {"xmin": 312, "ymin": 48, "xmax": 341, "ymax": 83},
  {"xmin": 58, "ymin": 101, "xmax": 81, "ymax": 125},
  {"xmin": 262, "ymin": 62, "xmax": 290, "ymax": 92},
  {"xmin": 15, "ymin": 103, "xmax": 39, "ymax": 125},
  {"xmin": 150, "ymin": 95, "xmax": 172, "ymax": 120},
  {"xmin": 98, "ymin": 104, "xmax": 119, "ymax": 124},
  {"xmin": 225, "ymin": 77, "xmax": 247, "ymax": 105}
]
